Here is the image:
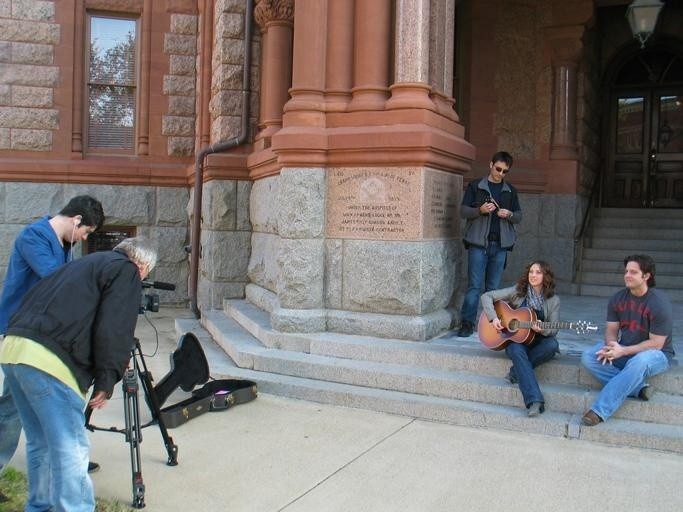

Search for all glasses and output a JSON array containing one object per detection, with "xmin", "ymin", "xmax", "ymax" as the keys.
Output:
[{"xmin": 493, "ymin": 164, "xmax": 509, "ymax": 173}]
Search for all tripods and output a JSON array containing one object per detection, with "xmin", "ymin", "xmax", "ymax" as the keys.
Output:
[{"xmin": 84, "ymin": 338, "xmax": 178, "ymax": 509}]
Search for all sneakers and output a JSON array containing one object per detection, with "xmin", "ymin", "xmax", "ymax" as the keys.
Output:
[{"xmin": 457, "ymin": 320, "xmax": 473, "ymax": 337}]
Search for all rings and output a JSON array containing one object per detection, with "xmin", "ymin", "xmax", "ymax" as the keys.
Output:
[{"xmin": 500, "ymin": 214, "xmax": 503, "ymax": 216}]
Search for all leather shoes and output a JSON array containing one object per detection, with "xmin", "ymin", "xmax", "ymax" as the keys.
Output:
[
  {"xmin": 582, "ymin": 409, "xmax": 602, "ymax": 426},
  {"xmin": 639, "ymin": 385, "xmax": 655, "ymax": 401}
]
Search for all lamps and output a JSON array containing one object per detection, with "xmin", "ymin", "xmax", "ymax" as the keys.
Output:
[{"xmin": 624, "ymin": 1, "xmax": 665, "ymax": 49}]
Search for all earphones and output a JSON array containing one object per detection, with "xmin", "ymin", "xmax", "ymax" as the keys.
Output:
[{"xmin": 74, "ymin": 219, "xmax": 81, "ymax": 225}]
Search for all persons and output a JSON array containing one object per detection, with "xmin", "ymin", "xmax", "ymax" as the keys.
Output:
[
  {"xmin": 478, "ymin": 260, "xmax": 562, "ymax": 418},
  {"xmin": 0, "ymin": 233, "xmax": 158, "ymax": 511},
  {"xmin": 575, "ymin": 252, "xmax": 676, "ymax": 428},
  {"xmin": 0, "ymin": 193, "xmax": 107, "ymax": 475},
  {"xmin": 453, "ymin": 149, "xmax": 524, "ymax": 337}
]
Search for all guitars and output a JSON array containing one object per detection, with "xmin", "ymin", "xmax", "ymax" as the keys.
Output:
[{"xmin": 477, "ymin": 300, "xmax": 598, "ymax": 350}]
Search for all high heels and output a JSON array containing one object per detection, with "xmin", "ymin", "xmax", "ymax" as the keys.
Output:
[
  {"xmin": 504, "ymin": 372, "xmax": 517, "ymax": 384},
  {"xmin": 527, "ymin": 401, "xmax": 545, "ymax": 417}
]
